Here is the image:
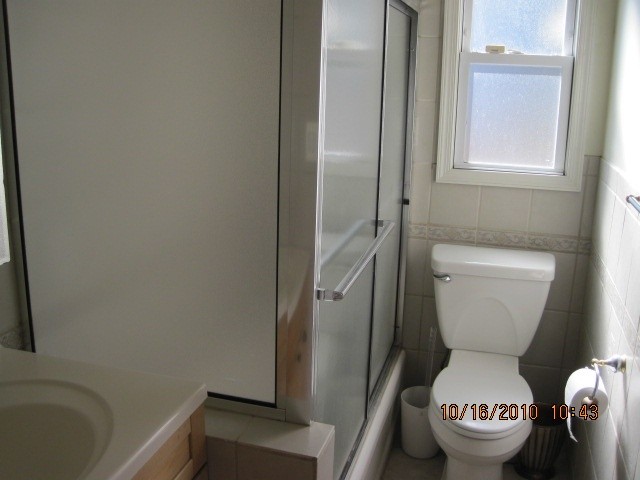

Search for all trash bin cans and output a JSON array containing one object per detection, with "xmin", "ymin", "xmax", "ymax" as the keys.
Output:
[
  {"xmin": 401, "ymin": 387, "xmax": 440, "ymax": 459},
  {"xmin": 513, "ymin": 401, "xmax": 566, "ymax": 480}
]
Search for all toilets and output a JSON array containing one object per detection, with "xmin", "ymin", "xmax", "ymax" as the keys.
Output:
[{"xmin": 426, "ymin": 243, "xmax": 556, "ymax": 479}]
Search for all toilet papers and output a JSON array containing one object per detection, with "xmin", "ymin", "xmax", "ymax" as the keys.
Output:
[{"xmin": 565, "ymin": 368, "xmax": 609, "ymax": 443}]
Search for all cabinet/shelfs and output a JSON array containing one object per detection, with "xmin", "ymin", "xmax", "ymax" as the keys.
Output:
[{"xmin": 129, "ymin": 400, "xmax": 211, "ymax": 480}]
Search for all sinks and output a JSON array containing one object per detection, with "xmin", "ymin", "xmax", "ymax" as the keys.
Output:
[{"xmin": 1, "ymin": 377, "xmax": 115, "ymax": 480}]
